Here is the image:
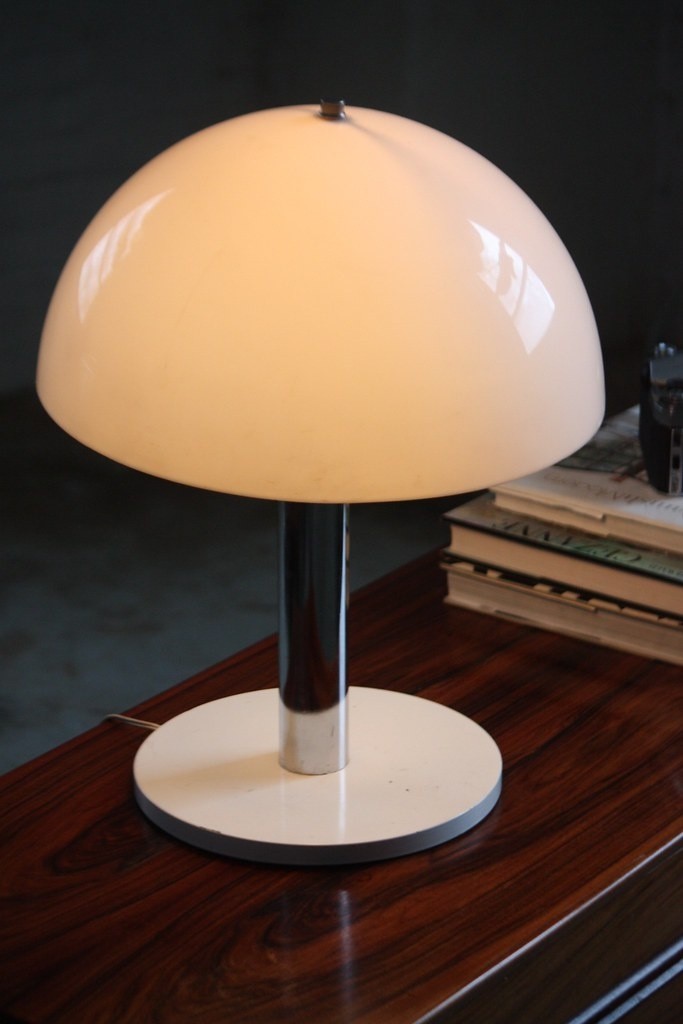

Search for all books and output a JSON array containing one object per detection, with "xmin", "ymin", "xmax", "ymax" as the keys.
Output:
[{"xmin": 441, "ymin": 402, "xmax": 683, "ymax": 664}]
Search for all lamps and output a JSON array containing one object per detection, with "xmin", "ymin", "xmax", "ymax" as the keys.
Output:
[{"xmin": 34, "ymin": 94, "xmax": 607, "ymax": 867}]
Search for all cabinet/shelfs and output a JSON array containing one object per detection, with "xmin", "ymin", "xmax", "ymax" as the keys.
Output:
[{"xmin": 0, "ymin": 543, "xmax": 683, "ymax": 1024}]
